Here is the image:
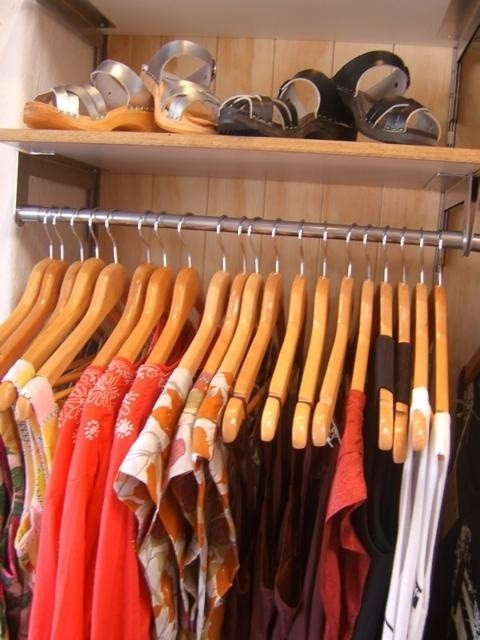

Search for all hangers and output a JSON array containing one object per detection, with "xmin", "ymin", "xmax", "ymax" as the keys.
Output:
[{"xmin": 0, "ymin": 208, "xmax": 451, "ymax": 463}]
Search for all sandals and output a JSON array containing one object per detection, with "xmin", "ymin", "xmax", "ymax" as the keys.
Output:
[
  {"xmin": 217, "ymin": 69, "xmax": 356, "ymax": 141},
  {"xmin": 331, "ymin": 51, "xmax": 442, "ymax": 146},
  {"xmin": 141, "ymin": 40, "xmax": 222, "ymax": 134},
  {"xmin": 24, "ymin": 60, "xmax": 169, "ymax": 132}
]
